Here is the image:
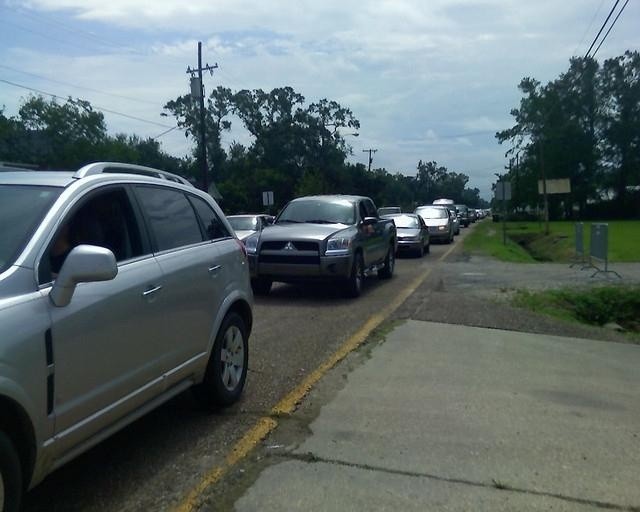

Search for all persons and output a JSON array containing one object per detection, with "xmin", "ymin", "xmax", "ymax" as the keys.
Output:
[{"xmin": 47, "ymin": 204, "xmax": 86, "ymax": 275}]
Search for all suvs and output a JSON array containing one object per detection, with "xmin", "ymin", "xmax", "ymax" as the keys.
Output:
[{"xmin": 0, "ymin": 160, "xmax": 254, "ymax": 512}]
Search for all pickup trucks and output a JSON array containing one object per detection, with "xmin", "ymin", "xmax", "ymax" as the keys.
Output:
[{"xmin": 245, "ymin": 195, "xmax": 397, "ymax": 298}]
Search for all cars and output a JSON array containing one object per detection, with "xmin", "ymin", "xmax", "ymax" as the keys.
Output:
[
  {"xmin": 377, "ymin": 199, "xmax": 492, "ymax": 258},
  {"xmin": 226, "ymin": 215, "xmax": 275, "ymax": 251}
]
